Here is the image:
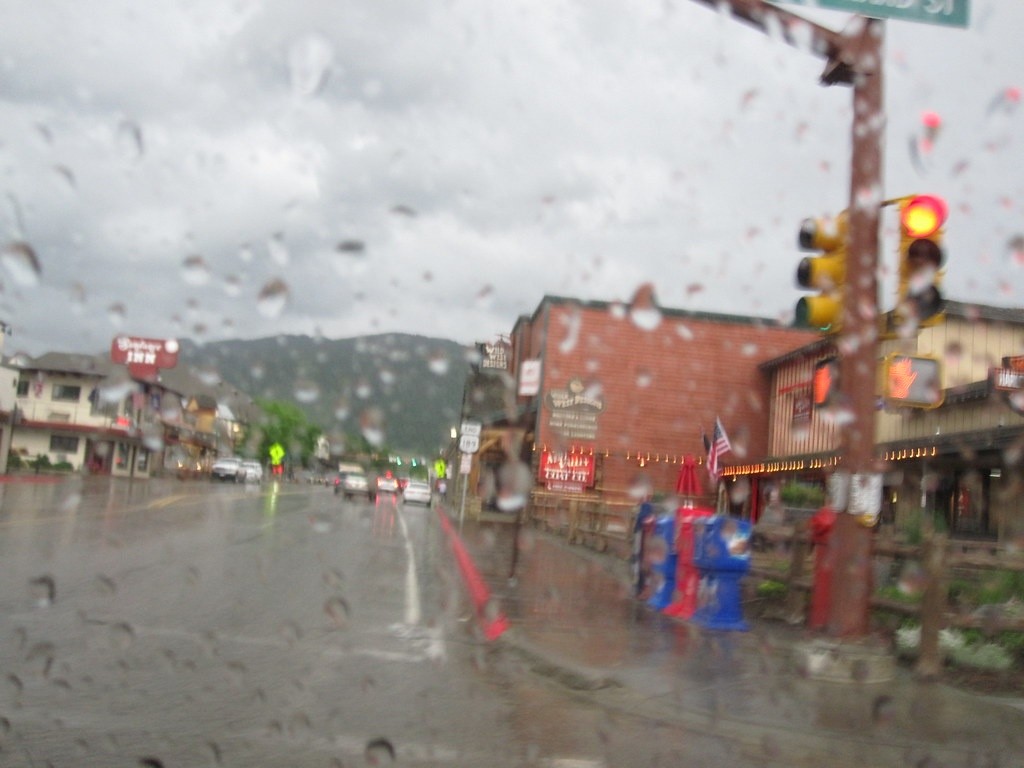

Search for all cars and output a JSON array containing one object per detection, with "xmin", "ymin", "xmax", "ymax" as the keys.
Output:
[
  {"xmin": 377, "ymin": 475, "xmax": 402, "ymax": 495},
  {"xmin": 335, "ymin": 472, "xmax": 373, "ymax": 503},
  {"xmin": 404, "ymin": 482, "xmax": 432, "ymax": 506},
  {"xmin": 244, "ymin": 463, "xmax": 264, "ymax": 486},
  {"xmin": 210, "ymin": 457, "xmax": 247, "ymax": 485}
]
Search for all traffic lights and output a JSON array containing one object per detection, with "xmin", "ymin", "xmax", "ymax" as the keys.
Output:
[
  {"xmin": 880, "ymin": 350, "xmax": 946, "ymax": 415},
  {"xmin": 811, "ymin": 353, "xmax": 846, "ymax": 409},
  {"xmin": 894, "ymin": 192, "xmax": 951, "ymax": 328},
  {"xmin": 793, "ymin": 210, "xmax": 858, "ymax": 337}
]
